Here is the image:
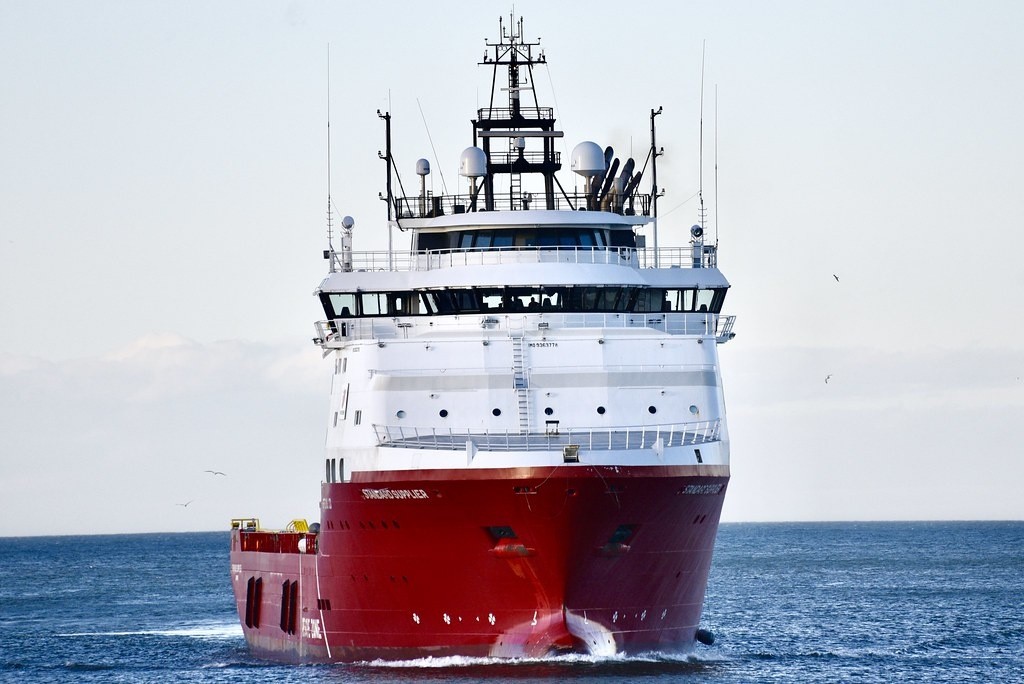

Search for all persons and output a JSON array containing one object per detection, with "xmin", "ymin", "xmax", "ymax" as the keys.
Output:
[{"xmin": 528, "ymin": 297, "xmax": 539, "ymax": 308}]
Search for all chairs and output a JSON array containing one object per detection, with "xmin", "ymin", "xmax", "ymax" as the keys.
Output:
[
  {"xmin": 341, "ymin": 307, "xmax": 350, "ymax": 315},
  {"xmin": 700, "ymin": 304, "xmax": 707, "ymax": 311},
  {"xmin": 518, "ymin": 298, "xmax": 523, "ymax": 308},
  {"xmin": 543, "ymin": 298, "xmax": 551, "ymax": 306}
]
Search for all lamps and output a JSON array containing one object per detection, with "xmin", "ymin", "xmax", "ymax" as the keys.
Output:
[
  {"xmin": 563, "ymin": 443, "xmax": 580, "ymax": 463},
  {"xmin": 538, "ymin": 322, "xmax": 549, "ymax": 329},
  {"xmin": 340, "ymin": 215, "xmax": 361, "ymax": 236},
  {"xmin": 690, "ymin": 224, "xmax": 703, "ymax": 247}
]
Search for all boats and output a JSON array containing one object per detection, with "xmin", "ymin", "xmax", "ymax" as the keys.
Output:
[{"xmin": 230, "ymin": 3, "xmax": 736, "ymax": 667}]
